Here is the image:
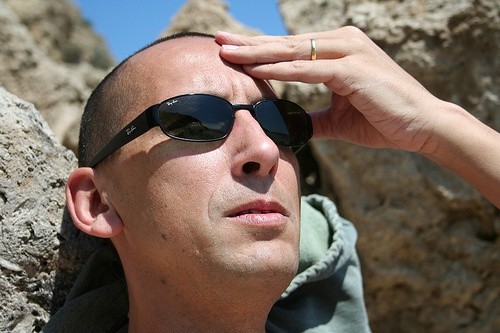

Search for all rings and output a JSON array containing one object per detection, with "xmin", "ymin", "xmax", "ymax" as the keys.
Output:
[{"xmin": 311, "ymin": 38, "xmax": 318, "ymax": 60}]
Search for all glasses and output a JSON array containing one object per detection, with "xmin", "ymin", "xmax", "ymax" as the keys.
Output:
[{"xmin": 89, "ymin": 93, "xmax": 314, "ymax": 167}]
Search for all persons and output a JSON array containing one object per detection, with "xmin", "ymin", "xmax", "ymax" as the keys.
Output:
[{"xmin": 40, "ymin": 31, "xmax": 500, "ymax": 333}]
